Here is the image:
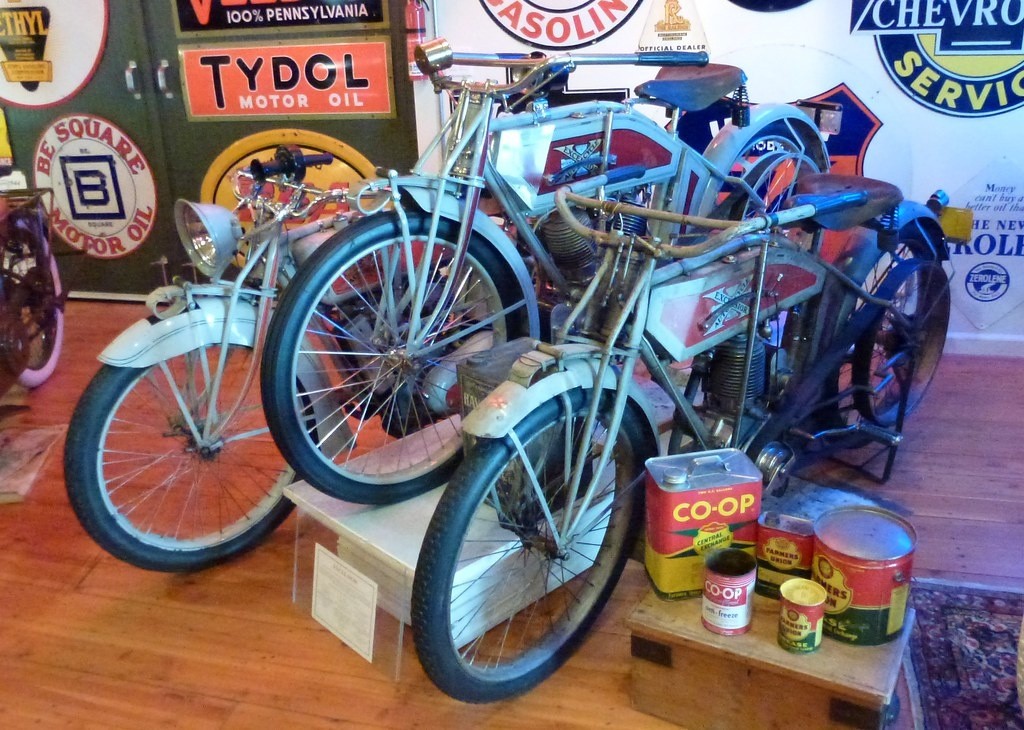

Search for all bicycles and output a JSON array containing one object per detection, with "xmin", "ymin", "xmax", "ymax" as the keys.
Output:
[
  {"xmin": 0, "ymin": 187, "xmax": 67, "ymax": 399},
  {"xmin": 412, "ymin": 175, "xmax": 953, "ymax": 705},
  {"xmin": 62, "ymin": 144, "xmax": 463, "ymax": 574},
  {"xmin": 260, "ymin": 38, "xmax": 830, "ymax": 503}
]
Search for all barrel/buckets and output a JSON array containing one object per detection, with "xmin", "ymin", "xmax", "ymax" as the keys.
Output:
[{"xmin": 812, "ymin": 497, "xmax": 920, "ymax": 646}]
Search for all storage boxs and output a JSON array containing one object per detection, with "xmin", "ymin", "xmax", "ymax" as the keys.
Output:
[{"xmin": 624, "ymin": 581, "xmax": 916, "ymax": 729}]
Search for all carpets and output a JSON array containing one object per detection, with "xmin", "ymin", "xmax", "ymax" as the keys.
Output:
[{"xmin": 901, "ymin": 577, "xmax": 1024, "ymax": 730}]
